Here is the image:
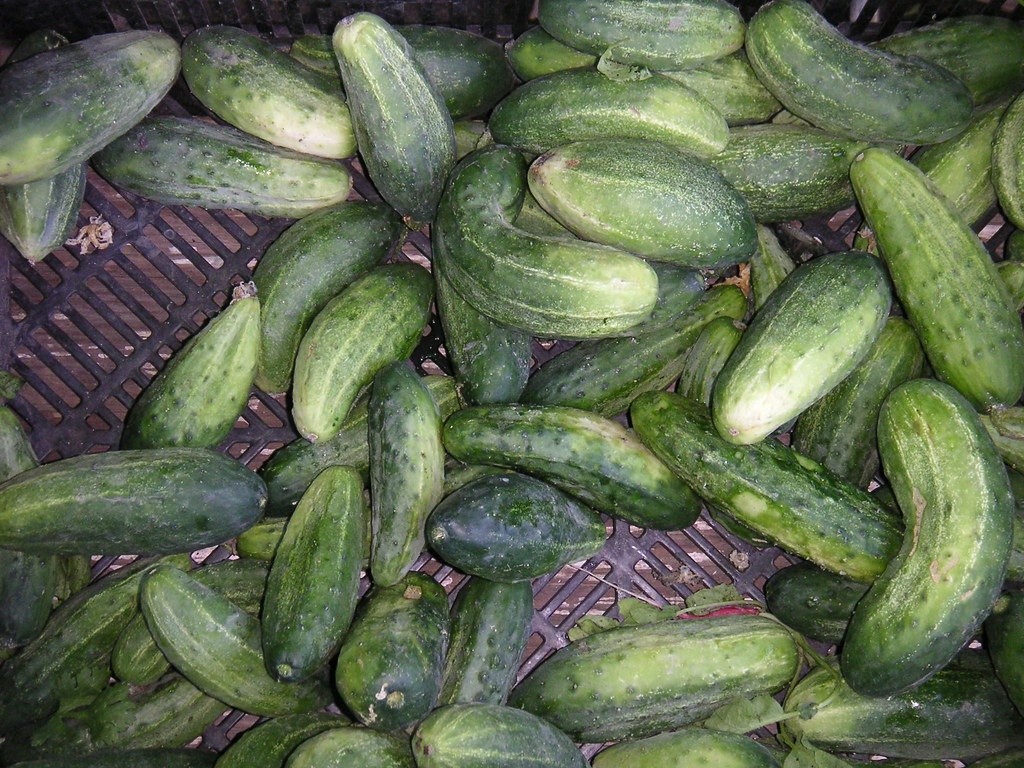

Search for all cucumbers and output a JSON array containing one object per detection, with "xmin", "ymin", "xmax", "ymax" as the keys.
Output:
[{"xmin": 0, "ymin": 0, "xmax": 1024, "ymax": 768}]
